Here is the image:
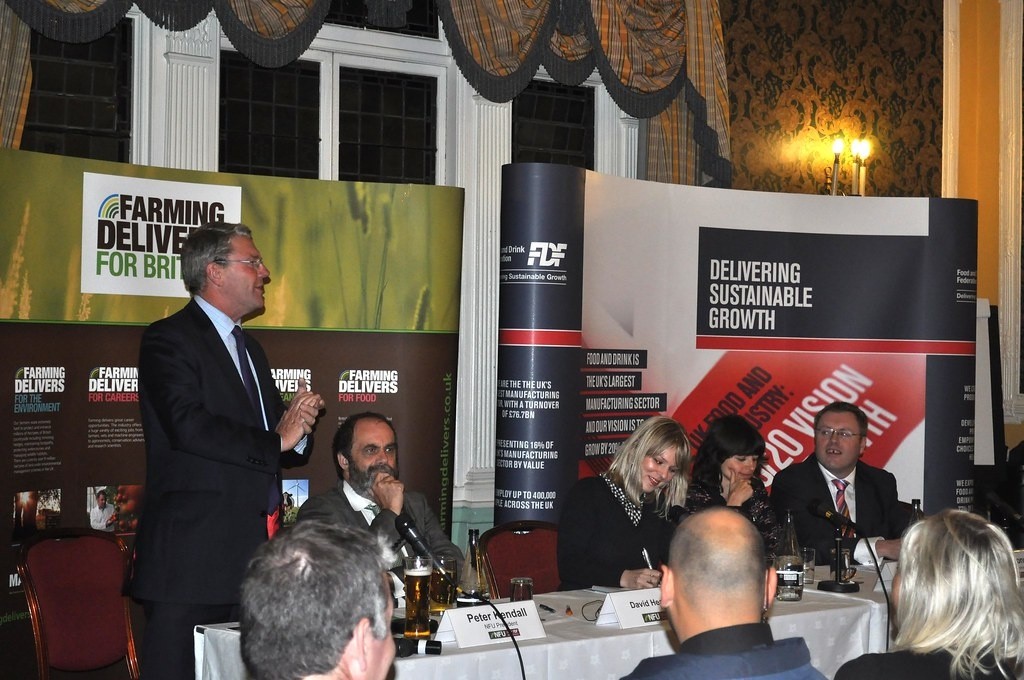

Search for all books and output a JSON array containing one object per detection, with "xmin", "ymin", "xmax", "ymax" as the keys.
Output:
[{"xmin": 592, "ymin": 586, "xmax": 632, "ymax": 593}]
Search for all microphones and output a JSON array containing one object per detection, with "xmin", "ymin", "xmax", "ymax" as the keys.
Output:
[
  {"xmin": 808, "ymin": 498, "xmax": 861, "ymax": 531},
  {"xmin": 395, "ymin": 513, "xmax": 462, "ymax": 592},
  {"xmin": 669, "ymin": 505, "xmax": 689, "ymax": 524},
  {"xmin": 392, "ymin": 638, "xmax": 442, "ymax": 658},
  {"xmin": 982, "ymin": 488, "xmax": 1023, "ymax": 522}
]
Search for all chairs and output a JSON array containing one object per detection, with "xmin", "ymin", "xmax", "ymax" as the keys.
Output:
[
  {"xmin": 15, "ymin": 528, "xmax": 144, "ymax": 680},
  {"xmin": 481, "ymin": 520, "xmax": 559, "ymax": 598}
]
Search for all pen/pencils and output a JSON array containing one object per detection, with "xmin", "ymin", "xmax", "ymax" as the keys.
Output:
[
  {"xmin": 539, "ymin": 603, "xmax": 555, "ymax": 614},
  {"xmin": 565, "ymin": 605, "xmax": 573, "ymax": 616},
  {"xmin": 641, "ymin": 547, "xmax": 653, "ymax": 568}
]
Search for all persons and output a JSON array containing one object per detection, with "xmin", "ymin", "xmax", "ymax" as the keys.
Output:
[
  {"xmin": 769, "ymin": 402, "xmax": 924, "ymax": 566},
  {"xmin": 121, "ymin": 221, "xmax": 326, "ymax": 680},
  {"xmin": 239, "ymin": 519, "xmax": 396, "ymax": 679},
  {"xmin": 619, "ymin": 507, "xmax": 829, "ymax": 680},
  {"xmin": 834, "ymin": 506, "xmax": 1023, "ymax": 680},
  {"xmin": 685, "ymin": 414, "xmax": 781, "ymax": 564},
  {"xmin": 295, "ymin": 411, "xmax": 466, "ymax": 609},
  {"xmin": 556, "ymin": 416, "xmax": 691, "ymax": 591},
  {"xmin": 90, "ymin": 491, "xmax": 118, "ymax": 532}
]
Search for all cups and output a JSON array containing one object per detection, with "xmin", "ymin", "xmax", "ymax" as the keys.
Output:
[
  {"xmin": 830, "ymin": 548, "xmax": 850, "ymax": 582},
  {"xmin": 800, "ymin": 548, "xmax": 815, "ymax": 584},
  {"xmin": 432, "ymin": 559, "xmax": 457, "ymax": 603},
  {"xmin": 403, "ymin": 556, "xmax": 432, "ymax": 642},
  {"xmin": 511, "ymin": 578, "xmax": 534, "ymax": 602},
  {"xmin": 1014, "ymin": 551, "xmax": 1024, "ymax": 579}
]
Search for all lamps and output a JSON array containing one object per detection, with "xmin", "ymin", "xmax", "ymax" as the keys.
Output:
[{"xmin": 821, "ymin": 138, "xmax": 872, "ymax": 197}]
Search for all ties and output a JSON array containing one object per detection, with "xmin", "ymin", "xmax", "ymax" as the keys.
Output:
[
  {"xmin": 232, "ymin": 324, "xmax": 266, "ymax": 431},
  {"xmin": 365, "ymin": 503, "xmax": 404, "ymax": 570},
  {"xmin": 832, "ymin": 478, "xmax": 854, "ymax": 538}
]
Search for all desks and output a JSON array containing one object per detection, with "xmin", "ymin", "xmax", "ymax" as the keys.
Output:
[{"xmin": 194, "ymin": 565, "xmax": 1024, "ymax": 680}]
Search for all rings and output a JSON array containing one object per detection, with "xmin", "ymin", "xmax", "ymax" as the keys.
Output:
[{"xmin": 650, "ymin": 574, "xmax": 654, "ymax": 582}]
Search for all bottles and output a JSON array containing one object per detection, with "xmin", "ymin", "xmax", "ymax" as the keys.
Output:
[
  {"xmin": 458, "ymin": 529, "xmax": 490, "ymax": 606},
  {"xmin": 1002, "ymin": 518, "xmax": 1010, "ymax": 536},
  {"xmin": 908, "ymin": 499, "xmax": 926, "ymax": 528},
  {"xmin": 775, "ymin": 509, "xmax": 804, "ymax": 601}
]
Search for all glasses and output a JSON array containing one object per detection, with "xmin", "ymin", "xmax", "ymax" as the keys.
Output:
[
  {"xmin": 212, "ymin": 258, "xmax": 263, "ymax": 271},
  {"xmin": 816, "ymin": 426, "xmax": 862, "ymax": 441}
]
